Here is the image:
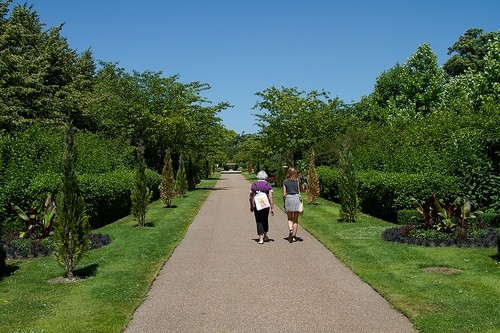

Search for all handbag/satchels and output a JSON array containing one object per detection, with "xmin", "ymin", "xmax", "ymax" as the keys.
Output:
[{"xmin": 299, "ymin": 199, "xmax": 303, "ymax": 215}]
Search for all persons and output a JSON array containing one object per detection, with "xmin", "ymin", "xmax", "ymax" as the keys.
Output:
[
  {"xmin": 282, "ymin": 167, "xmax": 302, "ymax": 243},
  {"xmin": 248, "ymin": 170, "xmax": 275, "ymax": 244}
]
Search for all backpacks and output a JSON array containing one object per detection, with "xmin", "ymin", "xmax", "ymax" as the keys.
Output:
[{"xmin": 254, "ymin": 190, "xmax": 271, "ymax": 211}]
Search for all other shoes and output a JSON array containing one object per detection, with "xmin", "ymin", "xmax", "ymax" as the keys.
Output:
[
  {"xmin": 263, "ymin": 236, "xmax": 269, "ymax": 241},
  {"xmin": 258, "ymin": 238, "xmax": 263, "ymax": 244},
  {"xmin": 288, "ymin": 230, "xmax": 298, "ymax": 243}
]
